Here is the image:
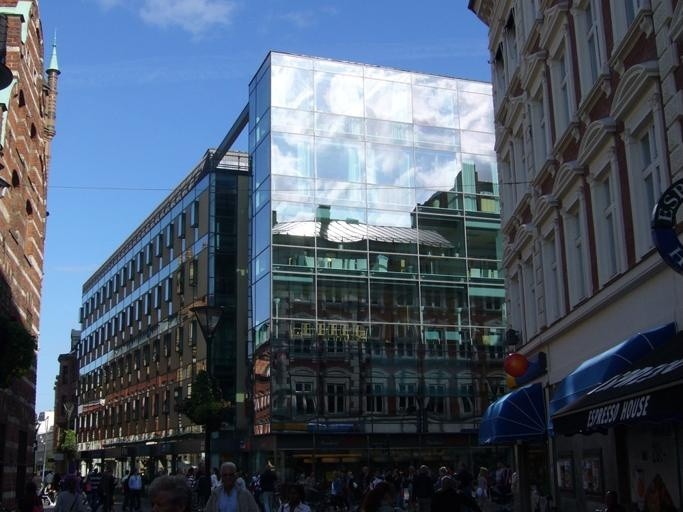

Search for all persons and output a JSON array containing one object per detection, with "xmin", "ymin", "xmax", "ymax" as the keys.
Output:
[
  {"xmin": 16, "ymin": 458, "xmax": 516, "ymax": 512},
  {"xmin": 529, "ymin": 478, "xmax": 553, "ymax": 511},
  {"xmin": 591, "ymin": 488, "xmax": 627, "ymax": 512}
]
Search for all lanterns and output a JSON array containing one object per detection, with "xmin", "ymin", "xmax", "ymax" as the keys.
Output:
[{"xmin": 502, "ymin": 352, "xmax": 529, "ymax": 379}]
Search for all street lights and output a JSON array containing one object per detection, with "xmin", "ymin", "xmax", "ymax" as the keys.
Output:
[{"xmin": 39, "ymin": 433, "xmax": 48, "ymax": 483}]
